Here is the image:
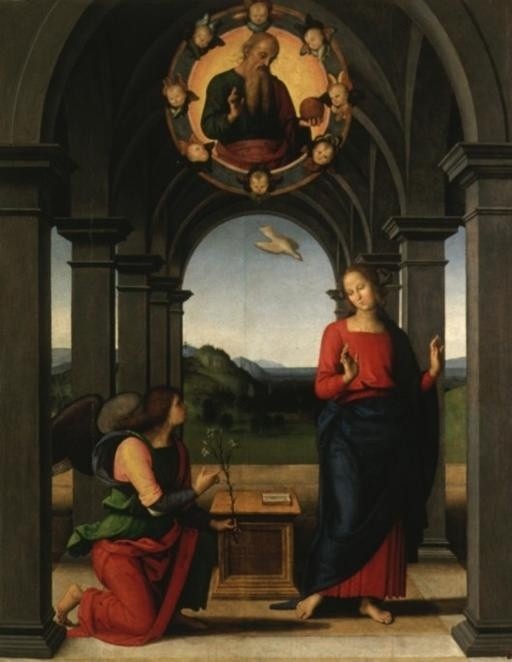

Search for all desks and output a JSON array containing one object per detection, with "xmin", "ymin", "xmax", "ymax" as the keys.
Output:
[{"xmin": 206, "ymin": 489, "xmax": 302, "ymax": 600}]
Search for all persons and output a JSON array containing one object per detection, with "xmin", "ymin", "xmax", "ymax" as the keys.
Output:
[
  {"xmin": 52, "ymin": 385, "xmax": 238, "ymax": 640},
  {"xmin": 295, "ymin": 262, "xmax": 445, "ymax": 626},
  {"xmin": 199, "ymin": 32, "xmax": 326, "ymax": 174}
]
